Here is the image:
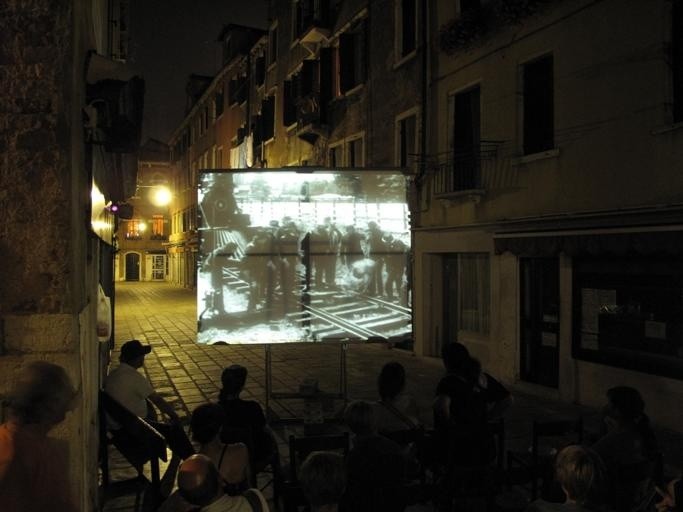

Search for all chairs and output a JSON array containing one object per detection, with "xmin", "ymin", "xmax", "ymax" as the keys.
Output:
[{"xmin": 98, "ymin": 388, "xmax": 683, "ymax": 512}]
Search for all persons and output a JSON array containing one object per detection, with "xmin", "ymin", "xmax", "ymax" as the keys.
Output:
[
  {"xmin": 104, "ymin": 339, "xmax": 195, "ymax": 461},
  {"xmin": 155, "ymin": 341, "xmax": 683, "ymax": 511},
  {"xmin": 240, "ymin": 215, "xmax": 413, "ymax": 315},
  {"xmin": 204, "ymin": 241, "xmax": 240, "ymax": 321},
  {"xmin": 0, "ymin": 358, "xmax": 71, "ymax": 512}
]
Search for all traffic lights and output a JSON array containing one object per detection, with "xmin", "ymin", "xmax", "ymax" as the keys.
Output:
[{"xmin": 110, "ymin": 203, "xmax": 119, "ymax": 213}]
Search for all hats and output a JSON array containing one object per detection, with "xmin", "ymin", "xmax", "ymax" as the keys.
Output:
[{"xmin": 120, "ymin": 340, "xmax": 152, "ymax": 357}]
